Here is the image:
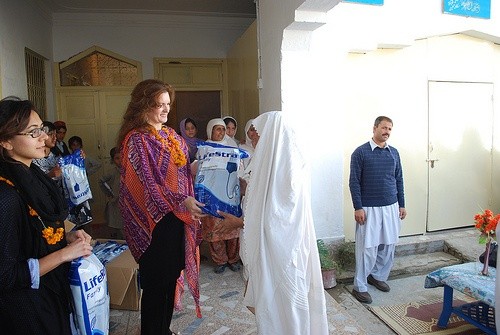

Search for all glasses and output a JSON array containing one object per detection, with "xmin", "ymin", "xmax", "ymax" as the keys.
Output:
[{"xmin": 15, "ymin": 126, "xmax": 49, "ymax": 138}]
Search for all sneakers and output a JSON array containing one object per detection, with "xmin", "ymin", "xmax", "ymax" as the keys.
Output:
[
  {"xmin": 367, "ymin": 274, "xmax": 390, "ymax": 291},
  {"xmin": 215, "ymin": 264, "xmax": 227, "ymax": 274},
  {"xmin": 228, "ymin": 263, "xmax": 243, "ymax": 272},
  {"xmin": 352, "ymin": 289, "xmax": 372, "ymax": 303}
]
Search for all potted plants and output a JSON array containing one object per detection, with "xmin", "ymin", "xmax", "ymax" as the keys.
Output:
[
  {"xmin": 340, "ymin": 238, "xmax": 355, "ymax": 272},
  {"xmin": 317, "ymin": 239, "xmax": 343, "ymax": 290}
]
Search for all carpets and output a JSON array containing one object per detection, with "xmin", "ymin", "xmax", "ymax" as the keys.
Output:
[{"xmin": 369, "ymin": 293, "xmax": 496, "ymax": 335}]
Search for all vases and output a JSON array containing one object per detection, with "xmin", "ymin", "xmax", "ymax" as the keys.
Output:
[{"xmin": 482, "ymin": 241, "xmax": 491, "ymax": 276}]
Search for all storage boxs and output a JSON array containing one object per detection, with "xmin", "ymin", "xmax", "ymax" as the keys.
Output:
[{"xmin": 90, "ymin": 238, "xmax": 141, "ymax": 311}]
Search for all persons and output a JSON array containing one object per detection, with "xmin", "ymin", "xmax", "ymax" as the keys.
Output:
[
  {"xmin": 197, "ymin": 118, "xmax": 245, "ymax": 274},
  {"xmin": 237, "ymin": 118, "xmax": 255, "ymax": 171},
  {"xmin": 222, "ymin": 115, "xmax": 240, "ymax": 145},
  {"xmin": 68, "ymin": 136, "xmax": 93, "ymax": 221},
  {"xmin": 349, "ymin": 116, "xmax": 407, "ymax": 304},
  {"xmin": 214, "ymin": 111, "xmax": 316, "ymax": 335},
  {"xmin": 118, "ymin": 79, "xmax": 211, "ymax": 335},
  {"xmin": 50, "ymin": 121, "xmax": 70, "ymax": 158},
  {"xmin": 180, "ymin": 117, "xmax": 204, "ymax": 164},
  {"xmin": 0, "ymin": 94, "xmax": 93, "ymax": 335},
  {"xmin": 99, "ymin": 146, "xmax": 125, "ymax": 240},
  {"xmin": 32, "ymin": 121, "xmax": 65, "ymax": 198}
]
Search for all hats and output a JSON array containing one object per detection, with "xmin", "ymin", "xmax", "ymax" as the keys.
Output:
[{"xmin": 54, "ymin": 120, "xmax": 66, "ymax": 128}]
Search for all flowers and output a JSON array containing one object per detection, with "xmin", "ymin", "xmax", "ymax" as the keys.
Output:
[{"xmin": 473, "ymin": 204, "xmax": 500, "ymax": 245}]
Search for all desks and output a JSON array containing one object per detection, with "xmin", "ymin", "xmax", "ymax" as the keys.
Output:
[{"xmin": 425, "ymin": 262, "xmax": 497, "ymax": 335}]
